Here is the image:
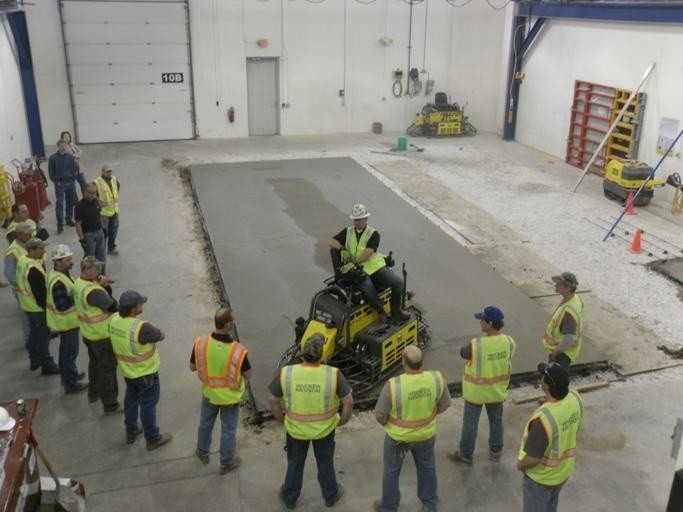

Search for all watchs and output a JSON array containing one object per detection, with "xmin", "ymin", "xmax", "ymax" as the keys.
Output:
[{"xmin": 339, "ymin": 246, "xmax": 346, "ymax": 251}]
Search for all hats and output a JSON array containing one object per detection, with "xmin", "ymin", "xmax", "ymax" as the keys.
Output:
[
  {"xmin": 12, "ymin": 220, "xmax": 107, "ymax": 269},
  {"xmin": 471, "ymin": 305, "xmax": 505, "ymax": 322},
  {"xmin": 118, "ymin": 287, "xmax": 148, "ymax": 307},
  {"xmin": 550, "ymin": 270, "xmax": 580, "ymax": 287},
  {"xmin": 101, "ymin": 163, "xmax": 113, "ymax": 172},
  {"xmin": 301, "ymin": 331, "xmax": 324, "ymax": 362},
  {"xmin": 533, "ymin": 359, "xmax": 571, "ymax": 387}
]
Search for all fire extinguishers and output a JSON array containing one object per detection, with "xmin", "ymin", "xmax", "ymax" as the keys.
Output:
[{"xmin": 228, "ymin": 106, "xmax": 235, "ymax": 122}]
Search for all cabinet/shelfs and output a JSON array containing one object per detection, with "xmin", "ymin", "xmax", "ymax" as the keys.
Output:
[{"xmin": 565, "ymin": 80, "xmax": 646, "ymax": 178}]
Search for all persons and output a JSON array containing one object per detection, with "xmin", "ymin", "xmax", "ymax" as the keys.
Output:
[
  {"xmin": 16, "ymin": 238, "xmax": 61, "ymax": 375},
  {"xmin": 267, "ymin": 334, "xmax": 354, "ymax": 509},
  {"xmin": 48, "ymin": 140, "xmax": 76, "ymax": 231},
  {"xmin": 5, "ymin": 203, "xmax": 37, "ymax": 243},
  {"xmin": 328, "ymin": 204, "xmax": 411, "ymax": 324},
  {"xmin": 43, "ymin": 244, "xmax": 89, "ymax": 392},
  {"xmin": 4, "ymin": 223, "xmax": 37, "ymax": 347},
  {"xmin": 516, "ymin": 362, "xmax": 585, "ymax": 512},
  {"xmin": 189, "ymin": 308, "xmax": 253, "ymax": 474},
  {"xmin": 91, "ymin": 164, "xmax": 120, "ymax": 255},
  {"xmin": 108, "ymin": 291, "xmax": 174, "ymax": 451},
  {"xmin": 74, "ymin": 184, "xmax": 106, "ymax": 269},
  {"xmin": 542, "ymin": 273, "xmax": 584, "ymax": 375},
  {"xmin": 60, "ymin": 131, "xmax": 87, "ymax": 191},
  {"xmin": 373, "ymin": 346, "xmax": 452, "ymax": 512},
  {"xmin": 72, "ymin": 256, "xmax": 125, "ymax": 414},
  {"xmin": 447, "ymin": 306, "xmax": 516, "ymax": 463}
]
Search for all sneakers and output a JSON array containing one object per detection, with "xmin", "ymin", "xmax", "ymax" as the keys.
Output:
[
  {"xmin": 124, "ymin": 424, "xmax": 144, "ymax": 445},
  {"xmin": 145, "ymin": 431, "xmax": 173, "ymax": 453},
  {"xmin": 389, "ymin": 303, "xmax": 411, "ymax": 321},
  {"xmin": 219, "ymin": 454, "xmax": 242, "ymax": 478},
  {"xmin": 57, "ymin": 219, "xmax": 122, "ymax": 256},
  {"xmin": 191, "ymin": 446, "xmax": 215, "ymax": 467},
  {"xmin": 275, "ymin": 484, "xmax": 298, "ymax": 510},
  {"xmin": 374, "ymin": 312, "xmax": 392, "ymax": 333},
  {"xmin": 443, "ymin": 449, "xmax": 503, "ymax": 468},
  {"xmin": 323, "ymin": 483, "xmax": 345, "ymax": 507},
  {"xmin": 370, "ymin": 498, "xmax": 399, "ymax": 511},
  {"xmin": 28, "ymin": 356, "xmax": 126, "ymax": 416}
]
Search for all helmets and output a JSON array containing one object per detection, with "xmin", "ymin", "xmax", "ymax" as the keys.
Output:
[{"xmin": 348, "ymin": 203, "xmax": 370, "ymax": 221}]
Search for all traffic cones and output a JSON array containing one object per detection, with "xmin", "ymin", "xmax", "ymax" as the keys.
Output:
[
  {"xmin": 627, "ymin": 229, "xmax": 643, "ymax": 255},
  {"xmin": 621, "ymin": 192, "xmax": 638, "ymax": 216}
]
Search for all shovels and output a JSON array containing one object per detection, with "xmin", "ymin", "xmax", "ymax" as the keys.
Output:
[{"xmin": 29, "ymin": 432, "xmax": 86, "ymax": 512}]
